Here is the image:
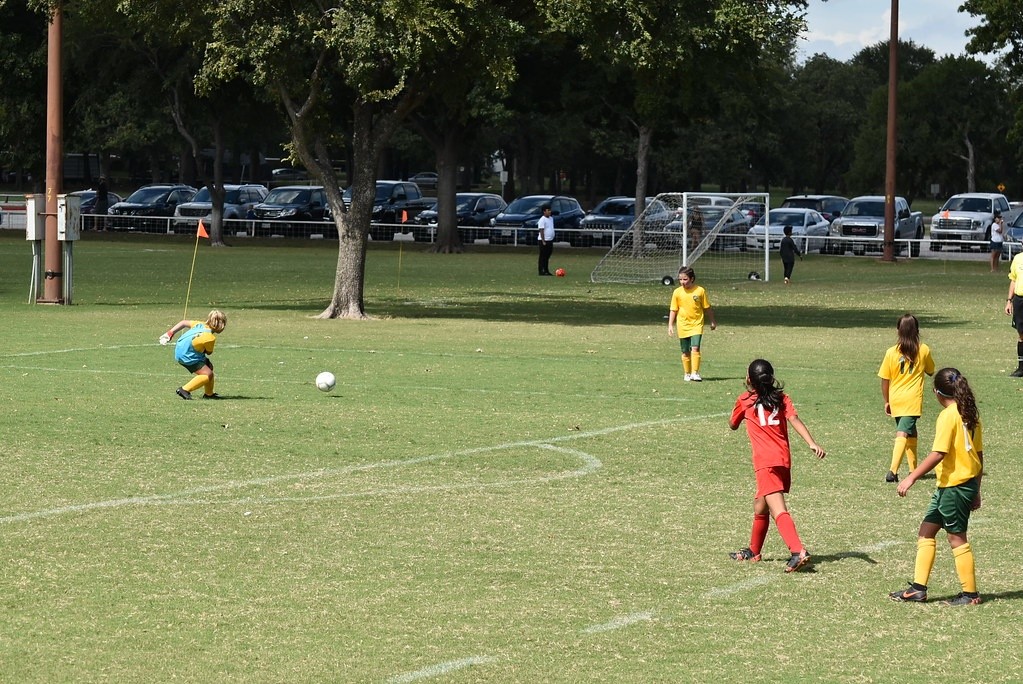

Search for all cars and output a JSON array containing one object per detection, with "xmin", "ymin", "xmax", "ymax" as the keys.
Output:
[
  {"xmin": 663, "ymin": 205, "xmax": 750, "ymax": 251},
  {"xmin": 72, "ymin": 190, "xmax": 126, "ymax": 230},
  {"xmin": 739, "ymin": 203, "xmax": 771, "ymax": 226},
  {"xmin": 746, "ymin": 208, "xmax": 830, "ymax": 254},
  {"xmin": 408, "ymin": 172, "xmax": 438, "ymax": 188},
  {"xmin": 1001, "ymin": 208, "xmax": 1023, "ymax": 261},
  {"xmin": 271, "ymin": 169, "xmax": 307, "ymax": 180}
]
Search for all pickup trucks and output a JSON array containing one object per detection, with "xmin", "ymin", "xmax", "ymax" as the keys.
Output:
[
  {"xmin": 829, "ymin": 196, "xmax": 925, "ymax": 258},
  {"xmin": 324, "ymin": 179, "xmax": 437, "ymax": 240}
]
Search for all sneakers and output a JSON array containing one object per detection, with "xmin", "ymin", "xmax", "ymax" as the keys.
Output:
[
  {"xmin": 683, "ymin": 374, "xmax": 691, "ymax": 382},
  {"xmin": 203, "ymin": 393, "xmax": 222, "ymax": 399},
  {"xmin": 690, "ymin": 373, "xmax": 702, "ymax": 381},
  {"xmin": 176, "ymin": 387, "xmax": 191, "ymax": 399},
  {"xmin": 888, "ymin": 582, "xmax": 927, "ymax": 602},
  {"xmin": 729, "ymin": 548, "xmax": 761, "ymax": 561},
  {"xmin": 784, "ymin": 549, "xmax": 811, "ymax": 572},
  {"xmin": 943, "ymin": 592, "xmax": 982, "ymax": 606}
]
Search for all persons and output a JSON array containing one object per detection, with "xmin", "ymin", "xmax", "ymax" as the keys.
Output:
[
  {"xmin": 160, "ymin": 309, "xmax": 227, "ymax": 400},
  {"xmin": 877, "ymin": 314, "xmax": 936, "ymax": 482},
  {"xmin": 668, "ymin": 267, "xmax": 717, "ymax": 381},
  {"xmin": 690, "ymin": 205, "xmax": 706, "ymax": 252},
  {"xmin": 537, "ymin": 205, "xmax": 555, "ymax": 276},
  {"xmin": 729, "ymin": 359, "xmax": 826, "ymax": 574},
  {"xmin": 888, "ymin": 368, "xmax": 983, "ymax": 607},
  {"xmin": 779, "ymin": 227, "xmax": 803, "ymax": 283},
  {"xmin": 991, "ymin": 214, "xmax": 1004, "ymax": 273},
  {"xmin": 91, "ymin": 174, "xmax": 108, "ymax": 230},
  {"xmin": 1005, "ymin": 252, "xmax": 1023, "ymax": 377}
]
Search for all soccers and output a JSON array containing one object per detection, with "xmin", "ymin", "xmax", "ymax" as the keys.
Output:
[
  {"xmin": 556, "ymin": 269, "xmax": 566, "ymax": 277},
  {"xmin": 315, "ymin": 371, "xmax": 336, "ymax": 392}
]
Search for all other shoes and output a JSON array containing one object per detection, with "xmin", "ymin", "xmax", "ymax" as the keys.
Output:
[
  {"xmin": 784, "ymin": 278, "xmax": 788, "ymax": 284},
  {"xmin": 1009, "ymin": 369, "xmax": 1023, "ymax": 377},
  {"xmin": 886, "ymin": 470, "xmax": 898, "ymax": 482}
]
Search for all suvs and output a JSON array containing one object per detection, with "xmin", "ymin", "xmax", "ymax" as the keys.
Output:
[
  {"xmin": 930, "ymin": 193, "xmax": 1011, "ymax": 251},
  {"xmin": 246, "ymin": 185, "xmax": 325, "ymax": 237},
  {"xmin": 781, "ymin": 194, "xmax": 850, "ymax": 223},
  {"xmin": 579, "ymin": 196, "xmax": 673, "ymax": 247},
  {"xmin": 106, "ymin": 183, "xmax": 198, "ymax": 232},
  {"xmin": 173, "ymin": 184, "xmax": 269, "ymax": 236},
  {"xmin": 489, "ymin": 194, "xmax": 585, "ymax": 245},
  {"xmin": 413, "ymin": 193, "xmax": 508, "ymax": 242}
]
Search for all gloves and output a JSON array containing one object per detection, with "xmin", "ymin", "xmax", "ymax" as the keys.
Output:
[{"xmin": 159, "ymin": 331, "xmax": 173, "ymax": 346}]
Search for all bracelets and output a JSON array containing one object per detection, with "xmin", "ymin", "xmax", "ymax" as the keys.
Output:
[{"xmin": 1007, "ymin": 299, "xmax": 1011, "ymax": 301}]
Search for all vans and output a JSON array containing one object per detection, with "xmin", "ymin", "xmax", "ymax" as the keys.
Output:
[{"xmin": 687, "ymin": 196, "xmax": 734, "ymax": 206}]
